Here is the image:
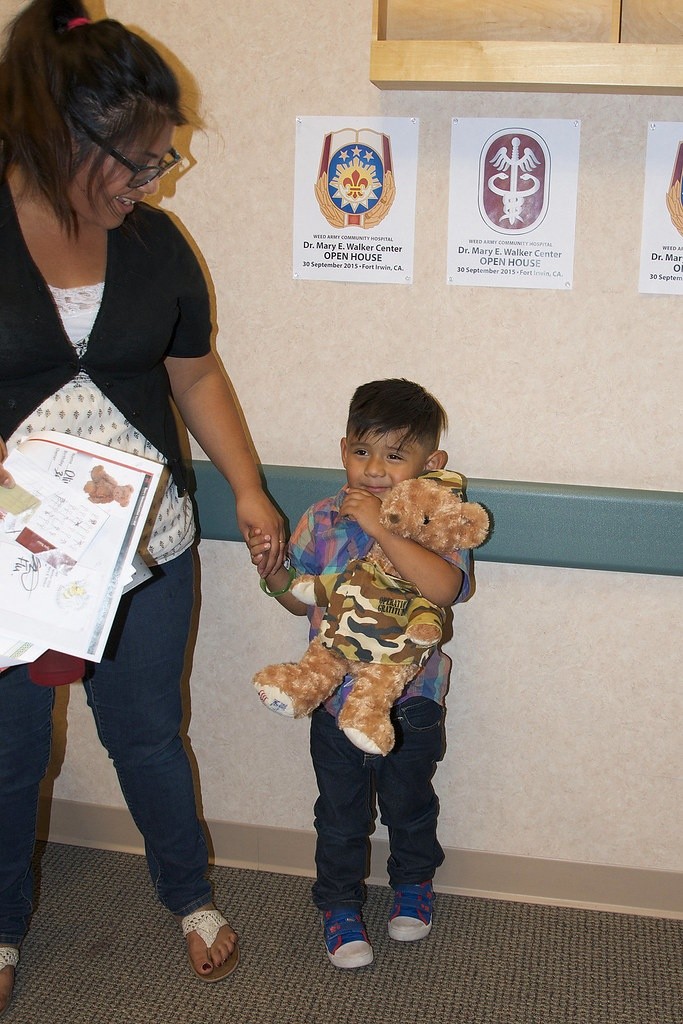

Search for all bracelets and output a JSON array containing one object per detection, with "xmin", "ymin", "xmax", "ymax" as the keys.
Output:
[{"xmin": 258, "ymin": 566, "xmax": 297, "ymax": 598}]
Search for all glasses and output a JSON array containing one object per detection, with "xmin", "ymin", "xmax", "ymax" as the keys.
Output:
[{"xmin": 61, "ymin": 104, "xmax": 183, "ymax": 188}]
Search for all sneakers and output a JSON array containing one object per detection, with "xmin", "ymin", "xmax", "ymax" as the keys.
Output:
[
  {"xmin": 321, "ymin": 905, "xmax": 374, "ymax": 969},
  {"xmin": 386, "ymin": 879, "xmax": 436, "ymax": 942}
]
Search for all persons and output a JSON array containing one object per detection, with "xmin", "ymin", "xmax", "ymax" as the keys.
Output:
[
  {"xmin": 247, "ymin": 378, "xmax": 480, "ymax": 969},
  {"xmin": 0, "ymin": 0, "xmax": 287, "ymax": 1017}
]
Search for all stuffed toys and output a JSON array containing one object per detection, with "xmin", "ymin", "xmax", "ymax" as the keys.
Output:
[{"xmin": 251, "ymin": 470, "xmax": 489, "ymax": 758}]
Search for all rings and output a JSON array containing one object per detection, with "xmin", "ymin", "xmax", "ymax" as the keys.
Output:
[{"xmin": 278, "ymin": 540, "xmax": 286, "ymax": 543}]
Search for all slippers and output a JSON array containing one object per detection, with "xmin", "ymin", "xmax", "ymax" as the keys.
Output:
[
  {"xmin": 0, "ymin": 946, "xmax": 19, "ymax": 1020},
  {"xmin": 179, "ymin": 901, "xmax": 241, "ymax": 983}
]
